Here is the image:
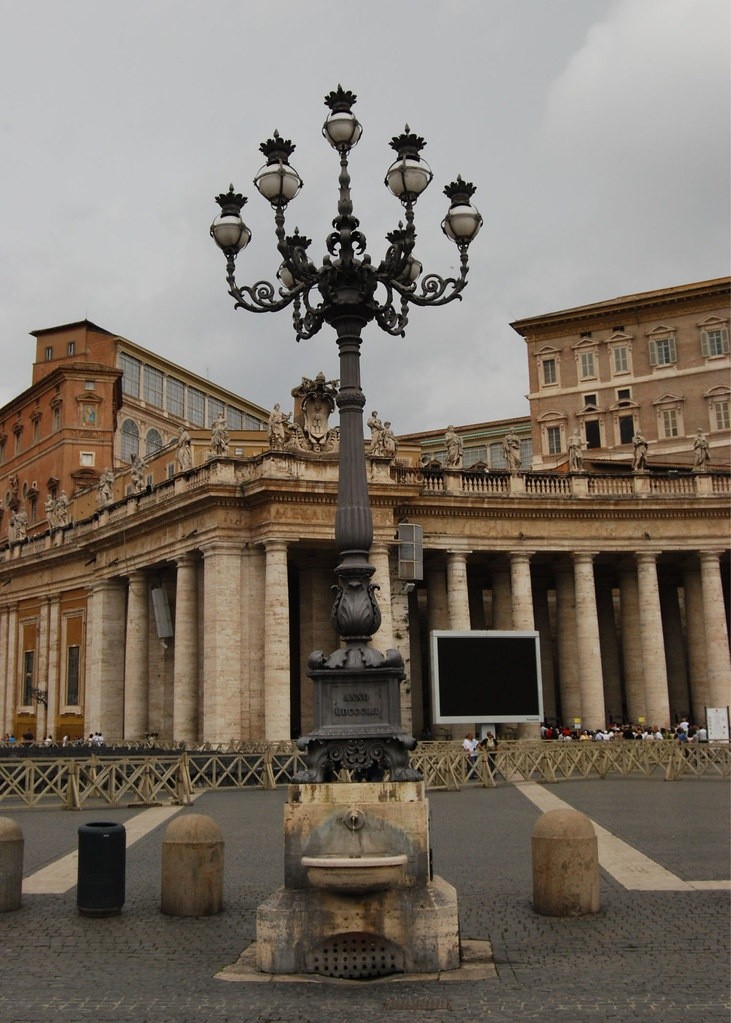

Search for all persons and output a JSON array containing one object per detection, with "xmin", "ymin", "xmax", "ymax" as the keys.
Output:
[
  {"xmin": 463, "ymin": 731, "xmax": 498, "ymax": 779},
  {"xmin": 55, "ymin": 490, "xmax": 67, "ymax": 523},
  {"xmin": 367, "ymin": 411, "xmax": 399, "ymax": 457},
  {"xmin": 100, "ymin": 468, "xmax": 114, "ymax": 503},
  {"xmin": 1, "ymin": 728, "xmax": 53, "ymax": 747},
  {"xmin": 692, "ymin": 429, "xmax": 711, "ymax": 471},
  {"xmin": 8, "ymin": 507, "xmax": 28, "ymax": 539},
  {"xmin": 63, "ymin": 732, "xmax": 69, "ymax": 746},
  {"xmin": 177, "ymin": 427, "xmax": 192, "ymax": 469},
  {"xmin": 88, "ymin": 732, "xmax": 104, "ymax": 747},
  {"xmin": 632, "ymin": 430, "xmax": 648, "ymax": 471},
  {"xmin": 568, "ymin": 429, "xmax": 589, "ymax": 470},
  {"xmin": 268, "ymin": 403, "xmax": 290, "ymax": 449},
  {"xmin": 145, "ymin": 732, "xmax": 158, "ymax": 745},
  {"xmin": 445, "ymin": 424, "xmax": 464, "ymax": 467},
  {"xmin": 43, "ymin": 494, "xmax": 56, "ymax": 527},
  {"xmin": 505, "ymin": 427, "xmax": 521, "ymax": 469},
  {"xmin": 211, "ymin": 411, "xmax": 230, "ymax": 455},
  {"xmin": 540, "ymin": 718, "xmax": 707, "ymax": 755},
  {"xmin": 130, "ymin": 452, "xmax": 145, "ymax": 492}
]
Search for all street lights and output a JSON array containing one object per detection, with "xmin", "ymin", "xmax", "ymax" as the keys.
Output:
[{"xmin": 210, "ymin": 86, "xmax": 485, "ymax": 784}]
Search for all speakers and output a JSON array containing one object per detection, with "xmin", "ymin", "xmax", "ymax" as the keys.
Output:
[
  {"xmin": 398, "ymin": 523, "xmax": 424, "ymax": 580},
  {"xmin": 151, "ymin": 588, "xmax": 174, "ymax": 639}
]
